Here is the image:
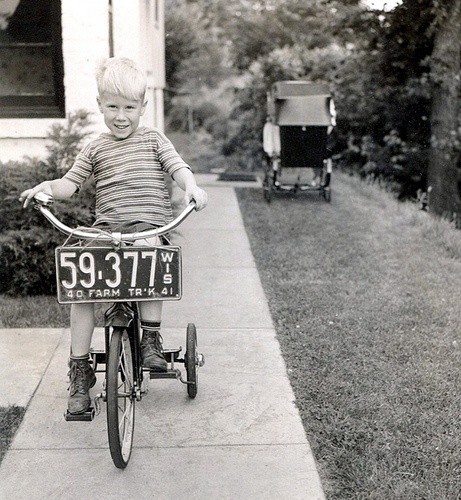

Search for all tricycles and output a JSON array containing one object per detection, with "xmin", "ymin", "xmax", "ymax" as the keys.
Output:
[
  {"xmin": 262, "ymin": 80, "xmax": 337, "ymax": 204},
  {"xmin": 31, "ymin": 191, "xmax": 205, "ymax": 470}
]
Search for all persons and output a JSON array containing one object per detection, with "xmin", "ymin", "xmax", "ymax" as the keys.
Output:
[{"xmin": 19, "ymin": 58, "xmax": 207, "ymax": 415}]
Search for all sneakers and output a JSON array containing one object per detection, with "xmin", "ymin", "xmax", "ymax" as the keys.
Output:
[
  {"xmin": 140, "ymin": 330, "xmax": 168, "ymax": 371},
  {"xmin": 67, "ymin": 358, "xmax": 96, "ymax": 415}
]
